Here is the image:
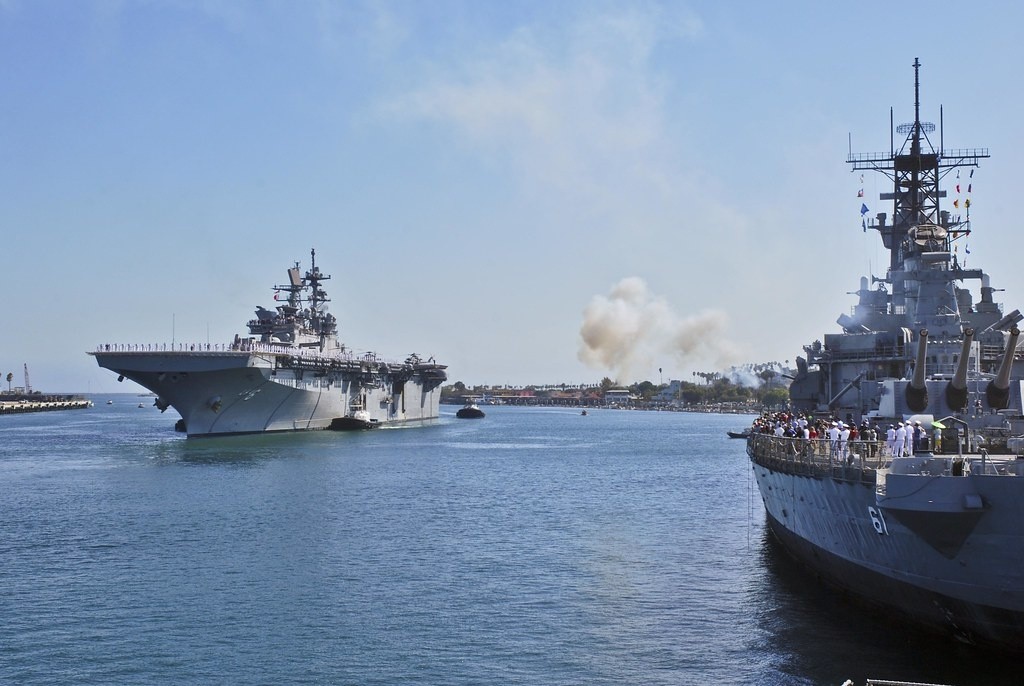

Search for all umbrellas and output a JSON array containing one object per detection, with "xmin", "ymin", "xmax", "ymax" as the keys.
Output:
[{"xmin": 931, "ymin": 421, "xmax": 946, "ymax": 429}]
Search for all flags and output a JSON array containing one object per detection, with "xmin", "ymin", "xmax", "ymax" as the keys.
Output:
[
  {"xmin": 953, "ymin": 170, "xmax": 960, "ymax": 254},
  {"xmin": 965, "ymin": 169, "xmax": 974, "ymax": 254},
  {"xmin": 857, "ymin": 174, "xmax": 869, "ymax": 232}
]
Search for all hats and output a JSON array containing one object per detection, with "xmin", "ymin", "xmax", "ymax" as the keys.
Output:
[
  {"xmin": 899, "ymin": 422, "xmax": 904, "ymax": 427},
  {"xmin": 843, "ymin": 424, "xmax": 850, "ymax": 428},
  {"xmin": 906, "ymin": 420, "xmax": 911, "ymax": 425},
  {"xmin": 832, "ymin": 422, "xmax": 838, "ymax": 426}
]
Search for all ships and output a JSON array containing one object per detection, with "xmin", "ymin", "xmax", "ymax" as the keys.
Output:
[
  {"xmin": 745, "ymin": 53, "xmax": 1024, "ymax": 661},
  {"xmin": 85, "ymin": 246, "xmax": 449, "ymax": 436}
]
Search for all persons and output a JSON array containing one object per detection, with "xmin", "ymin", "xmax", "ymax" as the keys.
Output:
[{"xmin": 751, "ymin": 409, "xmax": 942, "ymax": 461}]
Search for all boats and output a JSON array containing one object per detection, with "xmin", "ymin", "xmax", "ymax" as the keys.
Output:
[
  {"xmin": 331, "ymin": 409, "xmax": 383, "ymax": 431},
  {"xmin": 727, "ymin": 427, "xmax": 751, "ymax": 439},
  {"xmin": 456, "ymin": 396, "xmax": 486, "ymax": 420}
]
